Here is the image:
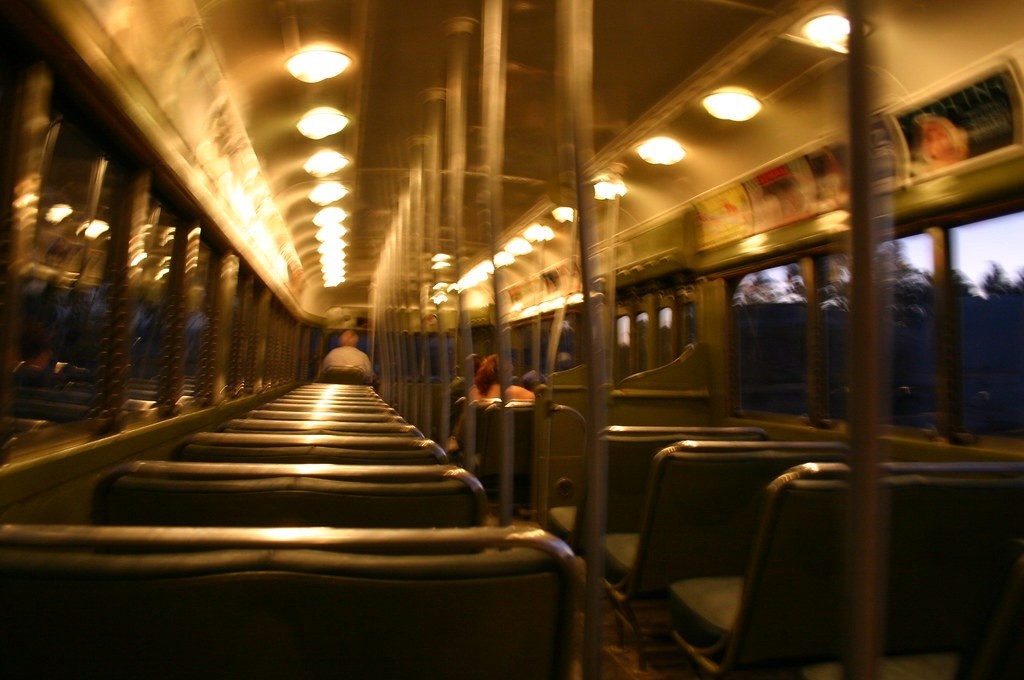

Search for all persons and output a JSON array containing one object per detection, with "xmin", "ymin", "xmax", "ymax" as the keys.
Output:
[
  {"xmin": 317, "ymin": 331, "xmax": 373, "ymax": 380},
  {"xmin": 449, "ymin": 354, "xmax": 535, "ymax": 412}
]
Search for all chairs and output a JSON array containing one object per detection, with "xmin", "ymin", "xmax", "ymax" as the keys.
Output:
[{"xmin": 318, "ymin": 365, "xmax": 366, "ymax": 385}]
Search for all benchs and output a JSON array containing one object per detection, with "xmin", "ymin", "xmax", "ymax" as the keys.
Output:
[{"xmin": 0, "ymin": 385, "xmax": 1023, "ymax": 680}]
